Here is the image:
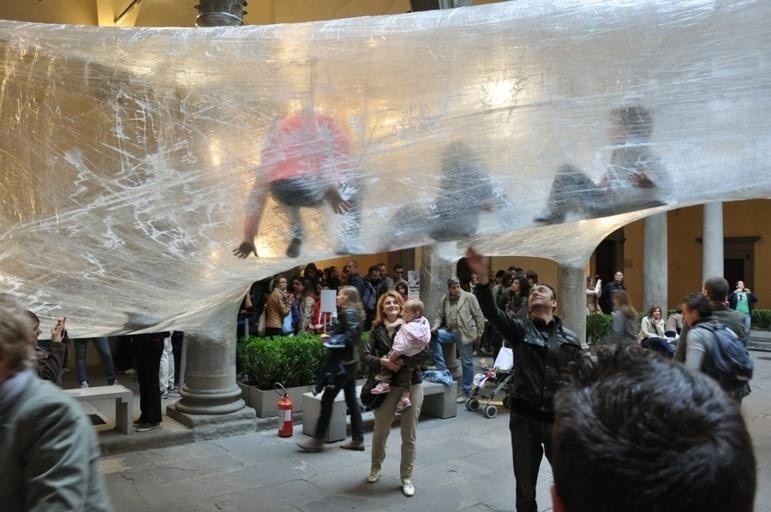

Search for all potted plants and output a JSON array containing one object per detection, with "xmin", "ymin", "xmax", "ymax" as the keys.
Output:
[{"xmin": 236, "ymin": 332, "xmax": 372, "ymax": 419}]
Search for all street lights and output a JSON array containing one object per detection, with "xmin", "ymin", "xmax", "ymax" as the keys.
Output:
[{"xmin": 166, "ymin": 0, "xmax": 258, "ymax": 428}]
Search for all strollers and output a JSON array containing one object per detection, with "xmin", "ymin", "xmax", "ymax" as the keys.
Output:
[{"xmin": 465, "ymin": 336, "xmax": 515, "ymax": 417}]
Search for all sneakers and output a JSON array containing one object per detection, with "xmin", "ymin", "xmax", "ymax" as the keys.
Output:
[
  {"xmin": 367, "ymin": 468, "xmax": 380, "ymax": 483},
  {"xmin": 400, "ymin": 476, "xmax": 415, "ymax": 497},
  {"xmin": 395, "ymin": 397, "xmax": 412, "ymax": 413},
  {"xmin": 369, "ymin": 381, "xmax": 392, "ymax": 395},
  {"xmin": 136, "ymin": 422, "xmax": 161, "ymax": 432}
]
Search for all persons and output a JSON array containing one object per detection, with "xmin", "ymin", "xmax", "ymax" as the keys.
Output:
[
  {"xmin": 374, "ymin": 129, "xmax": 502, "ymax": 246},
  {"xmin": 586, "ymin": 270, "xmax": 758, "ymax": 402},
  {"xmin": 550, "ymin": 343, "xmax": 756, "ymax": 512},
  {"xmin": 228, "ymin": 105, "xmax": 365, "ymax": 259},
  {"xmin": 527, "ymin": 94, "xmax": 674, "ymax": 230},
  {"xmin": 465, "ymin": 246, "xmax": 588, "ymax": 512},
  {"xmin": 1, "ymin": 295, "xmax": 113, "ymax": 511},
  {"xmin": 73, "ymin": 330, "xmax": 183, "ymax": 432},
  {"xmin": 19, "ymin": 307, "xmax": 73, "ymax": 390},
  {"xmin": 241, "ymin": 265, "xmax": 435, "ymax": 496},
  {"xmin": 435, "ymin": 267, "xmax": 538, "ymax": 395}
]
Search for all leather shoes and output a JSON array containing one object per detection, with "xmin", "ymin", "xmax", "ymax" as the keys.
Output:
[
  {"xmin": 296, "ymin": 437, "xmax": 322, "ymax": 451},
  {"xmin": 457, "ymin": 394, "xmax": 473, "ymax": 403},
  {"xmin": 340, "ymin": 440, "xmax": 366, "ymax": 450},
  {"xmin": 426, "ymin": 364, "xmax": 445, "ymax": 370}
]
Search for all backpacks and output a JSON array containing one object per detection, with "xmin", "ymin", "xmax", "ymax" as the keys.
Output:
[{"xmin": 696, "ymin": 320, "xmax": 753, "ymax": 390}]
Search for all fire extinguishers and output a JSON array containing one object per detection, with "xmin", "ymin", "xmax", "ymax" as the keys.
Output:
[{"xmin": 274, "ymin": 382, "xmax": 293, "ymax": 437}]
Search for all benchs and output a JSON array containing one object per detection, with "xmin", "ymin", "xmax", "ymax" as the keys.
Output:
[
  {"xmin": 54, "ymin": 381, "xmax": 136, "ymax": 436},
  {"xmin": 301, "ymin": 375, "xmax": 460, "ymax": 442}
]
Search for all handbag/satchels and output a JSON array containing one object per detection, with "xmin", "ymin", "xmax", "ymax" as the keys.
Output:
[
  {"xmin": 257, "ymin": 308, "xmax": 267, "ymax": 338},
  {"xmin": 360, "ymin": 377, "xmax": 387, "ymax": 413}
]
